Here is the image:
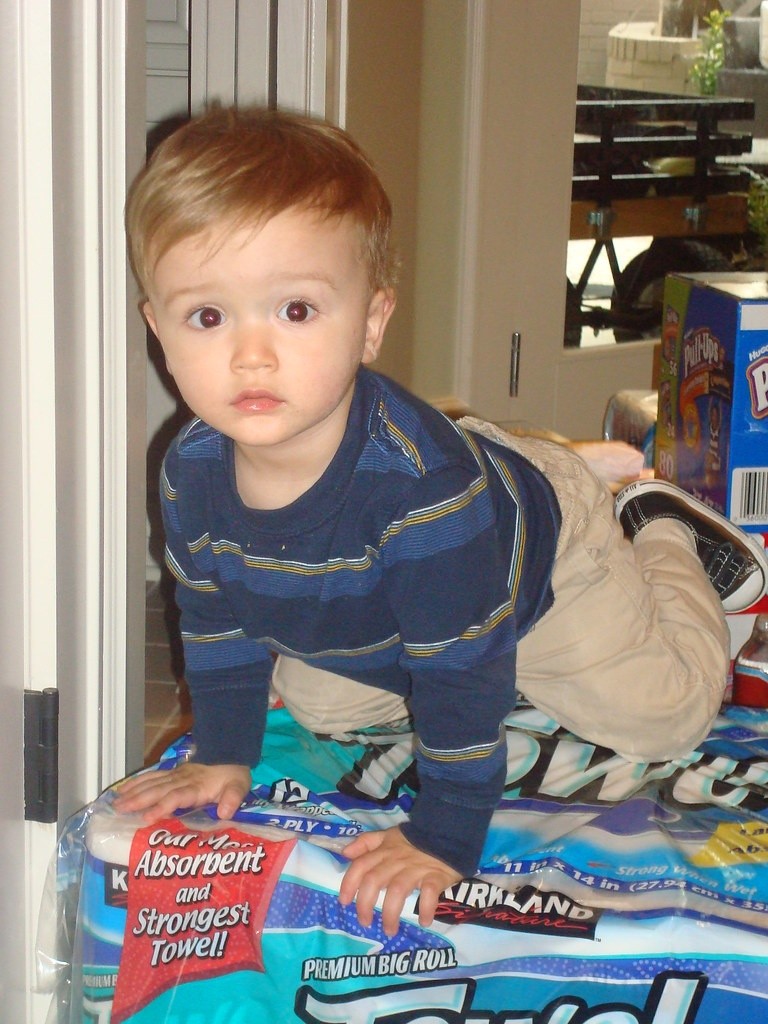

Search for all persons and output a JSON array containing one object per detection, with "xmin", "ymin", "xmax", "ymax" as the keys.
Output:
[
  {"xmin": 707, "ymin": 393, "xmax": 723, "ymax": 488},
  {"xmin": 112, "ymin": 105, "xmax": 768, "ymax": 937}
]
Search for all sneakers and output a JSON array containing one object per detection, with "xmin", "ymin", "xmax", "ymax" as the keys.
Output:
[{"xmin": 613, "ymin": 478, "xmax": 768, "ymax": 615}]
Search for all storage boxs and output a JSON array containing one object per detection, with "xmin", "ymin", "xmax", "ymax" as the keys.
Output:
[{"xmin": 654, "ymin": 273, "xmax": 768, "ymax": 617}]
